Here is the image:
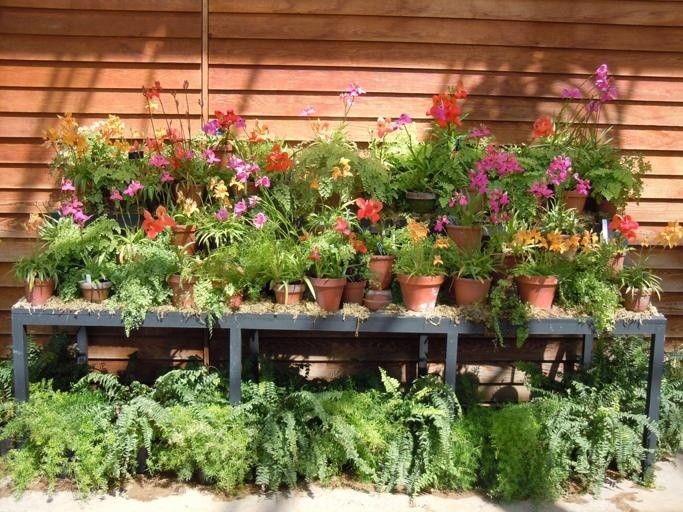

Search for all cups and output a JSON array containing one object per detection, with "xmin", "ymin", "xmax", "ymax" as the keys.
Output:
[{"xmin": 86, "ymin": 274, "xmax": 91, "ymax": 283}]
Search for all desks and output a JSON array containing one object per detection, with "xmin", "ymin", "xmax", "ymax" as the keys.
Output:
[{"xmin": 9, "ymin": 297, "xmax": 668, "ymax": 485}]
